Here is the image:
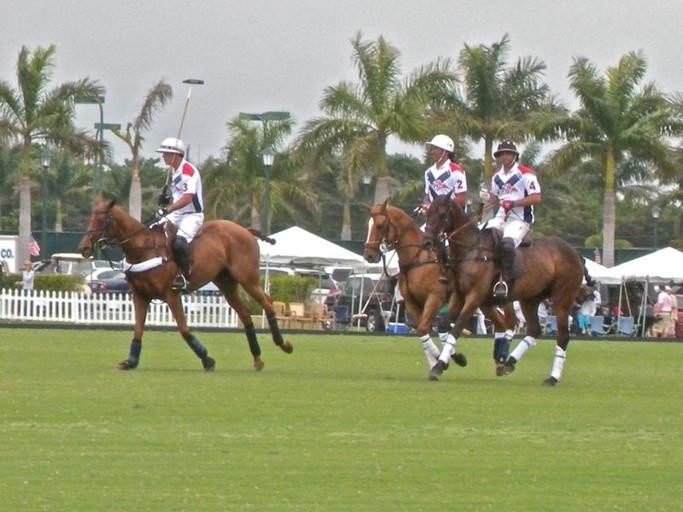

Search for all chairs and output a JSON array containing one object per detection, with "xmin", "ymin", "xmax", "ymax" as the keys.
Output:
[
  {"xmin": 588, "ymin": 315, "xmax": 607, "ymax": 335},
  {"xmin": 269, "ymin": 303, "xmax": 336, "ymax": 330},
  {"xmin": 616, "ymin": 315, "xmax": 638, "ymax": 337}
]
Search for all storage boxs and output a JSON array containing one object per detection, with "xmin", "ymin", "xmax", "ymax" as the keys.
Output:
[{"xmin": 388, "ymin": 323, "xmax": 409, "ymax": 333}]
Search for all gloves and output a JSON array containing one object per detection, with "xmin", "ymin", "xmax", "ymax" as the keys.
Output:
[
  {"xmin": 503, "ymin": 201, "xmax": 513, "ymax": 212},
  {"xmin": 151, "ymin": 208, "xmax": 164, "ymax": 220}
]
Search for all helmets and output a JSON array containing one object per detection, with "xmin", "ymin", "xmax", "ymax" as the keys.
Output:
[
  {"xmin": 425, "ymin": 134, "xmax": 455, "ymax": 154},
  {"xmin": 493, "ymin": 139, "xmax": 519, "ymax": 158},
  {"xmin": 154, "ymin": 138, "xmax": 185, "ymax": 157}
]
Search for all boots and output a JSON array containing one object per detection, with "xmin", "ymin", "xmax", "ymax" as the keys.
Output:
[
  {"xmin": 494, "ymin": 238, "xmax": 515, "ymax": 297},
  {"xmin": 172, "ymin": 236, "xmax": 189, "ymax": 286}
]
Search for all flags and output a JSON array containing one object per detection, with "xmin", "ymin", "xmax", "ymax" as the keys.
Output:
[{"xmin": 29, "ymin": 235, "xmax": 41, "ymax": 256}]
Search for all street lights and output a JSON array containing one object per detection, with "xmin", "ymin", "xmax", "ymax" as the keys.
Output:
[
  {"xmin": 238, "ymin": 109, "xmax": 290, "ymax": 239},
  {"xmin": 258, "ymin": 143, "xmax": 277, "ymax": 236},
  {"xmin": 649, "ymin": 203, "xmax": 661, "ymax": 251},
  {"xmin": 37, "ymin": 143, "xmax": 53, "ymax": 258},
  {"xmin": 89, "ymin": 118, "xmax": 122, "ymax": 210},
  {"xmin": 359, "ymin": 168, "xmax": 372, "ymax": 211}
]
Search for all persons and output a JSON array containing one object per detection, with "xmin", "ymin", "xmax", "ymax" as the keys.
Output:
[
  {"xmin": 411, "ymin": 133, "xmax": 469, "ymax": 246},
  {"xmin": 148, "ymin": 136, "xmax": 205, "ymax": 289},
  {"xmin": 15, "ymin": 261, "xmax": 35, "ymax": 315},
  {"xmin": 537, "ymin": 298, "xmax": 551, "ymax": 319},
  {"xmin": 476, "ymin": 142, "xmax": 543, "ymax": 299},
  {"xmin": 574, "ymin": 285, "xmax": 678, "ymax": 337}
]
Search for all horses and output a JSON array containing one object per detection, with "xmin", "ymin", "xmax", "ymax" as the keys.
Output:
[
  {"xmin": 419, "ymin": 184, "xmax": 594, "ymax": 388},
  {"xmin": 360, "ymin": 198, "xmax": 518, "ymax": 376},
  {"xmin": 76, "ymin": 192, "xmax": 293, "ymax": 373}
]
